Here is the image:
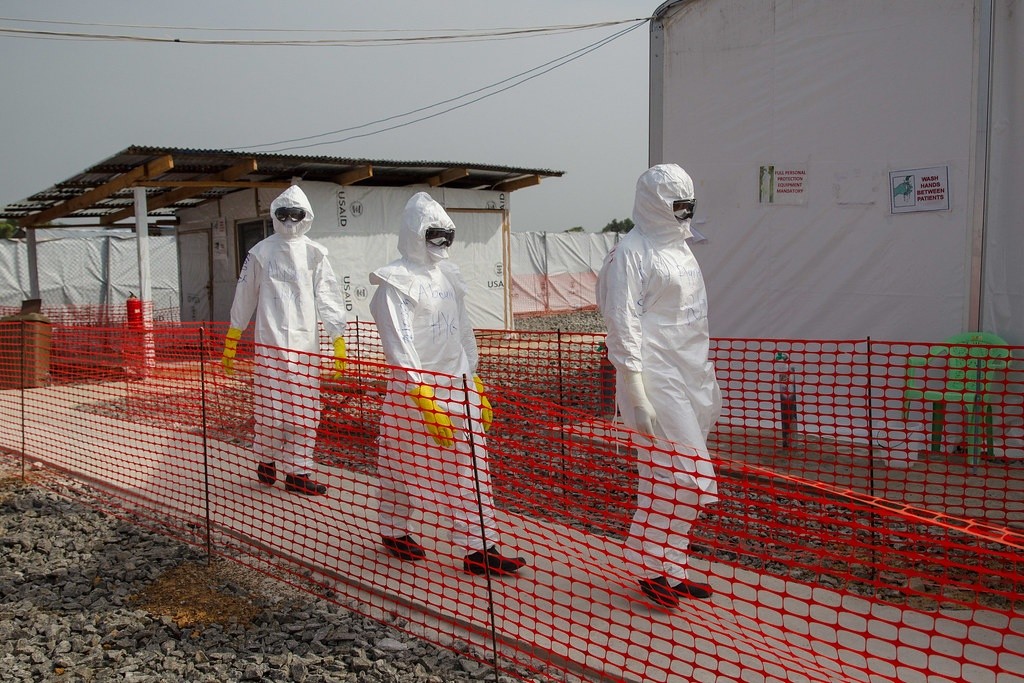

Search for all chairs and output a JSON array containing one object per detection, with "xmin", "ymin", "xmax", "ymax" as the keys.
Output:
[{"xmin": 903, "ymin": 333, "xmax": 1013, "ymax": 468}]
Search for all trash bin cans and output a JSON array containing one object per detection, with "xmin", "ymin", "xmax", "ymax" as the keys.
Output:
[{"xmin": 0, "ymin": 298, "xmax": 53, "ymax": 391}]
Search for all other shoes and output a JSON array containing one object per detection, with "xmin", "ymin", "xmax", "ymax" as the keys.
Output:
[
  {"xmin": 284, "ymin": 473, "xmax": 327, "ymax": 495},
  {"xmin": 381, "ymin": 534, "xmax": 426, "ymax": 560},
  {"xmin": 257, "ymin": 460, "xmax": 277, "ymax": 485},
  {"xmin": 671, "ymin": 578, "xmax": 713, "ymax": 598},
  {"xmin": 631, "ymin": 575, "xmax": 681, "ymax": 609},
  {"xmin": 463, "ymin": 545, "xmax": 527, "ymax": 576}
]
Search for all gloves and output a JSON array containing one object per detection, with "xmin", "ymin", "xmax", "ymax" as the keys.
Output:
[
  {"xmin": 332, "ymin": 336, "xmax": 347, "ymax": 380},
  {"xmin": 621, "ymin": 371, "xmax": 660, "ymax": 445},
  {"xmin": 221, "ymin": 328, "xmax": 243, "ymax": 375},
  {"xmin": 408, "ymin": 385, "xmax": 456, "ymax": 448},
  {"xmin": 473, "ymin": 376, "xmax": 493, "ymax": 431}
]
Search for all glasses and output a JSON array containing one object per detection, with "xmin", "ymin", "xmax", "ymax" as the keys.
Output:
[
  {"xmin": 673, "ymin": 198, "xmax": 697, "ymax": 220},
  {"xmin": 274, "ymin": 207, "xmax": 306, "ymax": 221},
  {"xmin": 425, "ymin": 227, "xmax": 455, "ymax": 248}
]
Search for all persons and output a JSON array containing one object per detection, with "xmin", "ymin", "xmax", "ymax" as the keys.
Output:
[
  {"xmin": 596, "ymin": 164, "xmax": 723, "ymax": 610},
  {"xmin": 220, "ymin": 184, "xmax": 346, "ymax": 497},
  {"xmin": 368, "ymin": 191, "xmax": 526, "ymax": 575}
]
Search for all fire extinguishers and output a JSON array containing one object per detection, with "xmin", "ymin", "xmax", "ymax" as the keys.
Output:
[{"xmin": 126, "ymin": 290, "xmax": 143, "ymax": 332}]
